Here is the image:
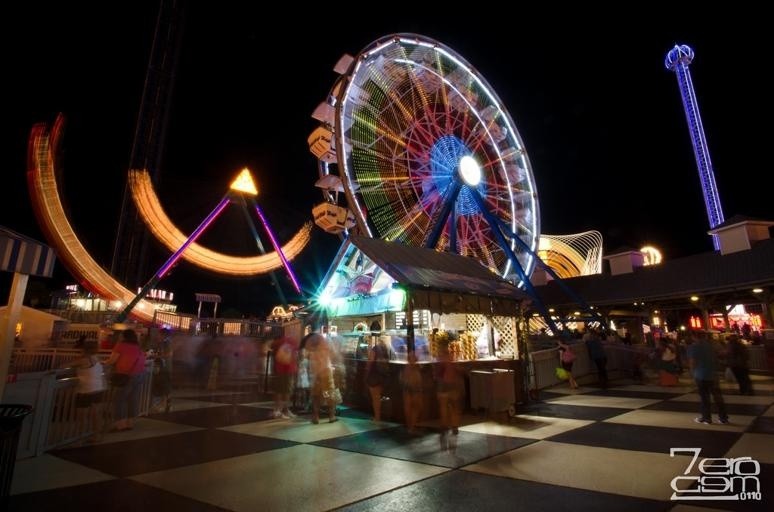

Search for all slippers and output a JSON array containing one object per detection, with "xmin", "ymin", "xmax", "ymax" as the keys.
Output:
[
  {"xmin": 328, "ymin": 416, "xmax": 338, "ymax": 422},
  {"xmin": 127, "ymin": 426, "xmax": 134, "ymax": 430},
  {"xmin": 313, "ymin": 418, "xmax": 320, "ymax": 425},
  {"xmin": 110, "ymin": 425, "xmax": 126, "ymax": 432}
]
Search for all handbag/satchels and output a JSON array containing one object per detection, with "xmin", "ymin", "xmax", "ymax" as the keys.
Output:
[
  {"xmin": 110, "ymin": 373, "xmax": 128, "ymax": 386},
  {"xmin": 366, "ymin": 361, "xmax": 384, "ymax": 388},
  {"xmin": 563, "ymin": 352, "xmax": 577, "ymax": 362}
]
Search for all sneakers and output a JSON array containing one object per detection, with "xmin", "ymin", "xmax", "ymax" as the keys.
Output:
[
  {"xmin": 716, "ymin": 419, "xmax": 730, "ymax": 424},
  {"xmin": 694, "ymin": 417, "xmax": 713, "ymax": 424},
  {"xmin": 273, "ymin": 409, "xmax": 280, "ymax": 417},
  {"xmin": 282, "ymin": 411, "xmax": 298, "ymax": 420}
]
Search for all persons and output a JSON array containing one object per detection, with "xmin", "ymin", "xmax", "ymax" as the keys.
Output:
[{"xmin": 57, "ymin": 321, "xmax": 759, "ymax": 463}]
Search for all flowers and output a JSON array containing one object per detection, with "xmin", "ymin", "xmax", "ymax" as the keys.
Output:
[{"xmin": 427, "ymin": 328, "xmax": 454, "ymax": 360}]
